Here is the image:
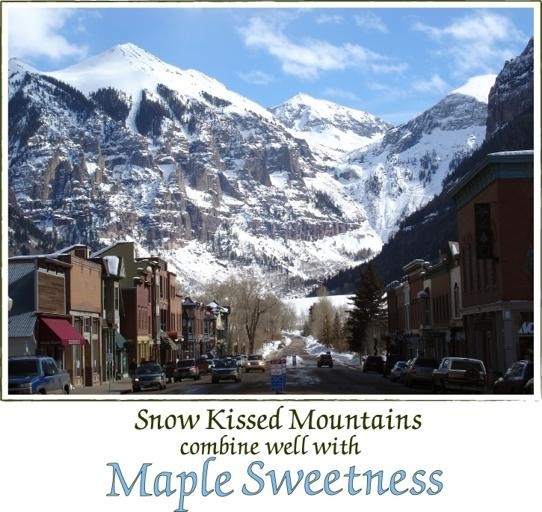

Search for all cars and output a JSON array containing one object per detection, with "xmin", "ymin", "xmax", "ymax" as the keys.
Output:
[
  {"xmin": 391, "ymin": 357, "xmax": 488, "ymax": 393},
  {"xmin": 132, "ymin": 363, "xmax": 166, "ymax": 391},
  {"xmin": 318, "ymin": 354, "xmax": 333, "ymax": 368},
  {"xmin": 166, "ymin": 354, "xmax": 266, "ymax": 382},
  {"xmin": 494, "ymin": 361, "xmax": 534, "ymax": 393},
  {"xmin": 363, "ymin": 356, "xmax": 385, "ymax": 373}
]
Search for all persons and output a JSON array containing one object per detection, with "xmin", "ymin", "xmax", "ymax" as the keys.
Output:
[{"xmin": 129, "ymin": 358, "xmax": 137, "ymax": 378}]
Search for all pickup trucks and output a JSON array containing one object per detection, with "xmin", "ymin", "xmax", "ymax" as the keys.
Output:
[{"xmin": 8, "ymin": 356, "xmax": 69, "ymax": 395}]
